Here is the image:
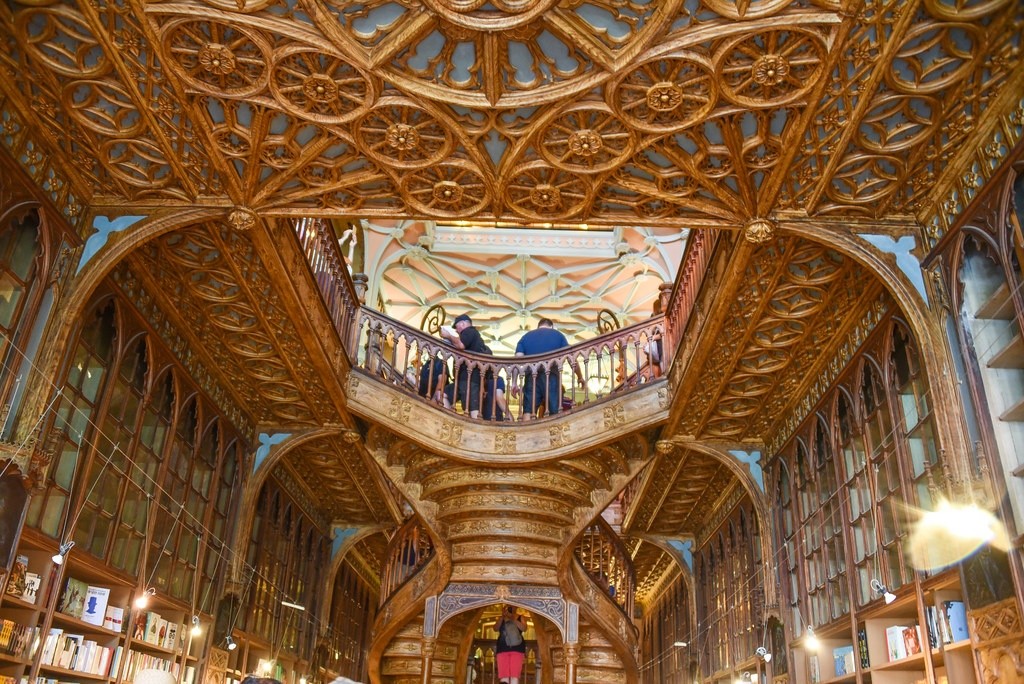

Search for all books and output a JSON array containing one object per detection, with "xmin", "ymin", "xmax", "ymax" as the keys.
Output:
[
  {"xmin": 132, "ymin": 610, "xmax": 191, "ymax": 654},
  {"xmin": 41, "ymin": 626, "xmax": 123, "ymax": 678},
  {"xmin": 833, "ymin": 629, "xmax": 870, "ymax": 677},
  {"xmin": 0, "ymin": 553, "xmax": 43, "ymax": 605},
  {"xmin": 122, "ymin": 647, "xmax": 195, "ymax": 683},
  {"xmin": 0, "ymin": 675, "xmax": 81, "ymax": 684},
  {"xmin": 57, "ymin": 578, "xmax": 124, "ymax": 633},
  {"xmin": 0, "ymin": 617, "xmax": 41, "ymax": 660},
  {"xmin": 809, "ymin": 656, "xmax": 819, "ymax": 684},
  {"xmin": 886, "ymin": 600, "xmax": 968, "ymax": 662},
  {"xmin": 226, "ymin": 658, "xmax": 322, "ymax": 684}
]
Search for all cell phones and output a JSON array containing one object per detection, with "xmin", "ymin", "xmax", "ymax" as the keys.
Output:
[{"xmin": 508, "ymin": 607, "xmax": 512, "ymax": 613}]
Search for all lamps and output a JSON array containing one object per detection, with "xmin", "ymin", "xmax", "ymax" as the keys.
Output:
[
  {"xmin": 224, "ymin": 636, "xmax": 236, "ymax": 650},
  {"xmin": 754, "ymin": 647, "xmax": 771, "ymax": 662},
  {"xmin": 52, "ymin": 540, "xmax": 75, "ymax": 565},
  {"xmin": 869, "ymin": 579, "xmax": 896, "ymax": 604},
  {"xmin": 190, "ymin": 615, "xmax": 202, "ymax": 635}
]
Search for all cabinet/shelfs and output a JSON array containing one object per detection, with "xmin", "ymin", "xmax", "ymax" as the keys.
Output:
[
  {"xmin": 225, "ymin": 628, "xmax": 298, "ymax": 684},
  {"xmin": 703, "ymin": 657, "xmax": 766, "ymax": 684},
  {"xmin": 296, "ymin": 658, "xmax": 338, "ymax": 684},
  {"xmin": 0, "ymin": 524, "xmax": 213, "ymax": 684},
  {"xmin": 788, "ymin": 569, "xmax": 978, "ymax": 684}
]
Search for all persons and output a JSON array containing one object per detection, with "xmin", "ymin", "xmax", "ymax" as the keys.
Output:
[
  {"xmin": 295, "ymin": 213, "xmax": 353, "ymax": 267},
  {"xmin": 595, "ymin": 571, "xmax": 616, "ymax": 598},
  {"xmin": 647, "ymin": 313, "xmax": 667, "ymax": 376},
  {"xmin": 393, "ymin": 519, "xmax": 417, "ymax": 584},
  {"xmin": 392, "ymin": 315, "xmax": 585, "ymax": 422},
  {"xmin": 493, "ymin": 604, "xmax": 527, "ymax": 684}
]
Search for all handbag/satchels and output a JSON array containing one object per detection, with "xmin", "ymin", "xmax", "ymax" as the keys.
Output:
[
  {"xmin": 644, "ymin": 340, "xmax": 659, "ymax": 363},
  {"xmin": 503, "ymin": 624, "xmax": 523, "ymax": 647}
]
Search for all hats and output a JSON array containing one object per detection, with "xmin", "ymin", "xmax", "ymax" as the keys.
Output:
[{"xmin": 452, "ymin": 314, "xmax": 471, "ymax": 329}]
